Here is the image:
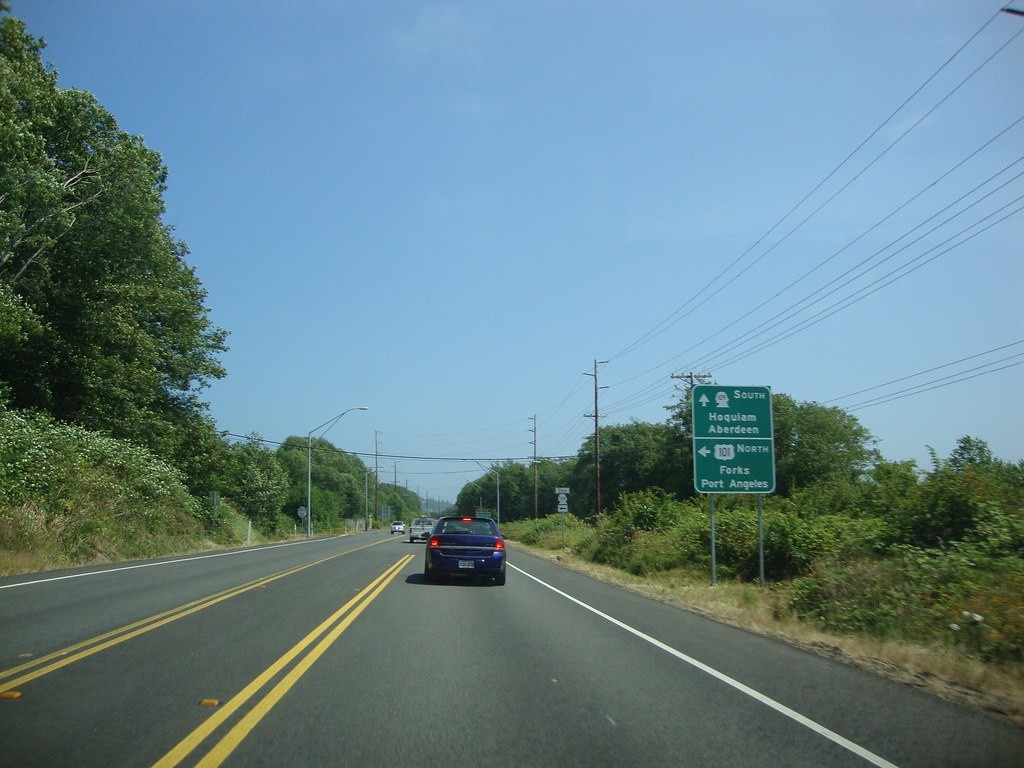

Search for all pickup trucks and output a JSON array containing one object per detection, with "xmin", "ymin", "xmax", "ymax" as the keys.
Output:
[{"xmin": 409, "ymin": 517, "xmax": 435, "ymax": 543}]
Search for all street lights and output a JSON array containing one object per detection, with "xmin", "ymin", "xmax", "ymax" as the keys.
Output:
[
  {"xmin": 364, "ymin": 465, "xmax": 384, "ymax": 531},
  {"xmin": 307, "ymin": 407, "xmax": 368, "ymax": 539},
  {"xmin": 475, "ymin": 460, "xmax": 500, "ymax": 534},
  {"xmin": 457, "ymin": 476, "xmax": 483, "ymax": 512}
]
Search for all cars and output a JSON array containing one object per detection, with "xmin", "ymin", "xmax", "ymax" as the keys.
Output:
[
  {"xmin": 425, "ymin": 516, "xmax": 506, "ymax": 587},
  {"xmin": 390, "ymin": 520, "xmax": 406, "ymax": 535}
]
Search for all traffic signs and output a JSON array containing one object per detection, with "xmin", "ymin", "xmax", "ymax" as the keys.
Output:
[{"xmin": 691, "ymin": 385, "xmax": 778, "ymax": 494}]
